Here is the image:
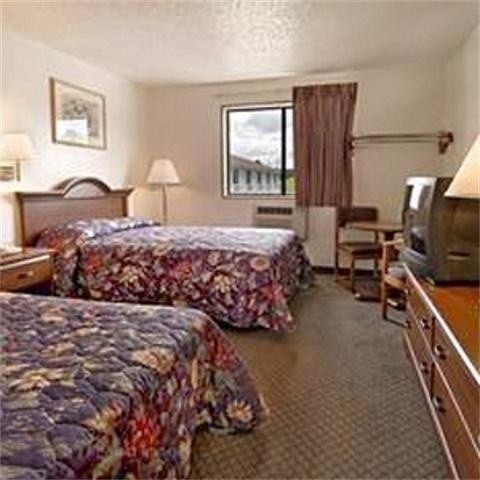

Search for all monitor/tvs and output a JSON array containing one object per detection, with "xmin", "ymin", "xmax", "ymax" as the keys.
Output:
[{"xmin": 403, "ymin": 174, "xmax": 480, "ymax": 285}]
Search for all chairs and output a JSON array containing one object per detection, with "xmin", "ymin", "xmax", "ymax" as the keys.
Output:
[{"xmin": 333, "ymin": 203, "xmax": 406, "ymax": 319}]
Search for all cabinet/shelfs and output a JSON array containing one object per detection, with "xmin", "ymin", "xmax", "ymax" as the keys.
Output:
[{"xmin": 402, "ymin": 261, "xmax": 480, "ymax": 480}]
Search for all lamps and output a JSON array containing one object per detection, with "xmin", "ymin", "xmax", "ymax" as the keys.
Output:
[
  {"xmin": 147, "ymin": 160, "xmax": 183, "ymax": 221},
  {"xmin": 0, "ymin": 134, "xmax": 34, "ymax": 182}
]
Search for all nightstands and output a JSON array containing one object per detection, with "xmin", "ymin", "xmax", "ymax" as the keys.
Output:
[{"xmin": 1, "ymin": 246, "xmax": 57, "ymax": 295}]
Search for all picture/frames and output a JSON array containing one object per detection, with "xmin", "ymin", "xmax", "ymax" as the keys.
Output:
[{"xmin": 50, "ymin": 77, "xmax": 106, "ymax": 151}]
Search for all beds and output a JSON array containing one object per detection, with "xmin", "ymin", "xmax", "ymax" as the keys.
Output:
[
  {"xmin": 15, "ymin": 176, "xmax": 317, "ymax": 333},
  {"xmin": 0, "ymin": 291, "xmax": 270, "ymax": 480}
]
